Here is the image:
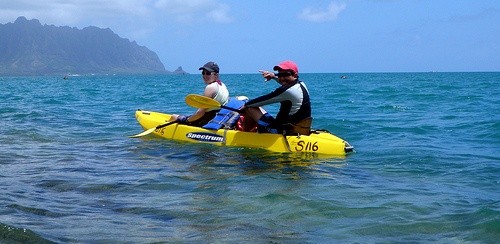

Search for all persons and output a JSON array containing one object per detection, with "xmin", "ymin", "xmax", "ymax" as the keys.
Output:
[
  {"xmin": 169, "ymin": 61, "xmax": 229, "ymax": 127},
  {"xmin": 239, "ymin": 63, "xmax": 312, "ymax": 135}
]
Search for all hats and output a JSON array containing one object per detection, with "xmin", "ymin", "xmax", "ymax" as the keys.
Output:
[
  {"xmin": 273, "ymin": 62, "xmax": 298, "ymax": 75},
  {"xmin": 199, "ymin": 62, "xmax": 219, "ymax": 73}
]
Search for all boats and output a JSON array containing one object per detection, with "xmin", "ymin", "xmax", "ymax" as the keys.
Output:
[{"xmin": 135, "ymin": 109, "xmax": 354, "ymax": 156}]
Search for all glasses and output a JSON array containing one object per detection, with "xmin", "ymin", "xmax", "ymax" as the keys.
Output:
[
  {"xmin": 276, "ymin": 72, "xmax": 292, "ymax": 77},
  {"xmin": 202, "ymin": 71, "xmax": 216, "ymax": 76}
]
